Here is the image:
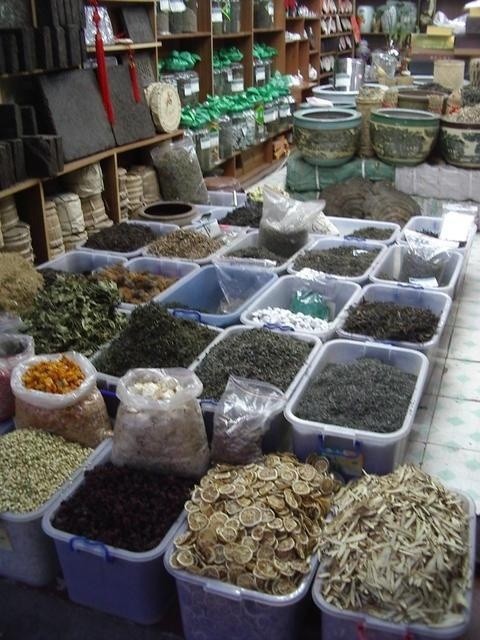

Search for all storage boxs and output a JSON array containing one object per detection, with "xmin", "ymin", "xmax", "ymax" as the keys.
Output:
[{"xmin": 0, "ymin": 193, "xmax": 478, "ymax": 640}]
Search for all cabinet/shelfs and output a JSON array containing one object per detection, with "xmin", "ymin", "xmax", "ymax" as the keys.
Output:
[
  {"xmin": 0, "ymin": 0, "xmax": 186, "ymax": 258},
  {"xmin": 155, "ymin": 0, "xmax": 356, "ymax": 193}
]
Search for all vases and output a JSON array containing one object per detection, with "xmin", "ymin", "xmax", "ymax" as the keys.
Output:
[{"xmin": 357, "ymin": 2, "xmax": 417, "ymax": 67}]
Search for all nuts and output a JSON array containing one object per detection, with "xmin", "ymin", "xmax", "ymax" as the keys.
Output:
[{"xmin": 169, "ymin": 450, "xmax": 342, "ymax": 640}]
